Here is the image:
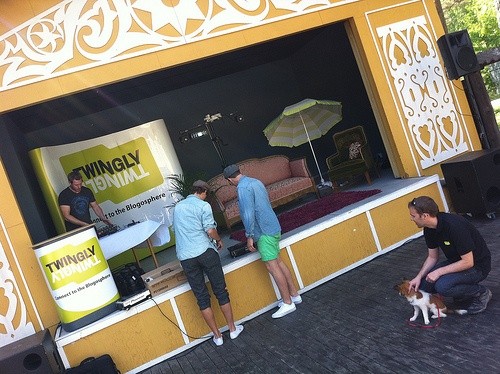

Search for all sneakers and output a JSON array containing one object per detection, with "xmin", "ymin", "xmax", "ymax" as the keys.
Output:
[
  {"xmin": 230, "ymin": 325, "xmax": 244, "ymax": 339},
  {"xmin": 271, "ymin": 302, "xmax": 296, "ymax": 318},
  {"xmin": 213, "ymin": 335, "xmax": 223, "ymax": 346},
  {"xmin": 470, "ymin": 289, "xmax": 492, "ymax": 314},
  {"xmin": 278, "ymin": 294, "xmax": 302, "ymax": 308}
]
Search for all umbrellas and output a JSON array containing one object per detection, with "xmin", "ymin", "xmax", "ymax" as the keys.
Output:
[{"xmin": 263, "ymin": 96, "xmax": 343, "ymax": 187}]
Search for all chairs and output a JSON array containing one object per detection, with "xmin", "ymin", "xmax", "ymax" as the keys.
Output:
[{"xmin": 325, "ymin": 125, "xmax": 380, "ymax": 192}]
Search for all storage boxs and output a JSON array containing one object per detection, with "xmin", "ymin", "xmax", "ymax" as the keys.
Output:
[{"xmin": 141, "ymin": 260, "xmax": 188, "ymax": 297}]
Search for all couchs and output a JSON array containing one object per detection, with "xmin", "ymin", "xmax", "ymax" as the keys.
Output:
[{"xmin": 205, "ymin": 154, "xmax": 321, "ymax": 231}]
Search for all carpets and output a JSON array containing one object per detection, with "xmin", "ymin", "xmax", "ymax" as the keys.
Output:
[{"xmin": 230, "ymin": 189, "xmax": 382, "ymax": 242}]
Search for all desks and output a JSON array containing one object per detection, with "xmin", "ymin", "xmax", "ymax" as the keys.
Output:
[{"xmin": 100, "ymin": 220, "xmax": 159, "ymax": 269}]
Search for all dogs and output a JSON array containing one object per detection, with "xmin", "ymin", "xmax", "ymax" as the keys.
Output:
[{"xmin": 392, "ymin": 277, "xmax": 468, "ymax": 326}]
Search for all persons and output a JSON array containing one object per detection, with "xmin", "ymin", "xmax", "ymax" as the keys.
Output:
[
  {"xmin": 173, "ymin": 180, "xmax": 245, "ymax": 346},
  {"xmin": 224, "ymin": 164, "xmax": 302, "ymax": 319},
  {"xmin": 57, "ymin": 172, "xmax": 112, "ymax": 234},
  {"xmin": 408, "ymin": 195, "xmax": 492, "ymax": 314}
]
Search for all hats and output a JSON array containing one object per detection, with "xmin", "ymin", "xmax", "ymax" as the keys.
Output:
[
  {"xmin": 192, "ymin": 180, "xmax": 210, "ymax": 190},
  {"xmin": 223, "ymin": 164, "xmax": 240, "ymax": 179}
]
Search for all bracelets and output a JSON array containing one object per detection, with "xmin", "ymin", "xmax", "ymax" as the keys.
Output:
[{"xmin": 217, "ymin": 238, "xmax": 221, "ymax": 242}]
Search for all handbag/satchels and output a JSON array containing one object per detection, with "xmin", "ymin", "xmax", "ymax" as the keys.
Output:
[{"xmin": 64, "ymin": 353, "xmax": 121, "ymax": 374}]
